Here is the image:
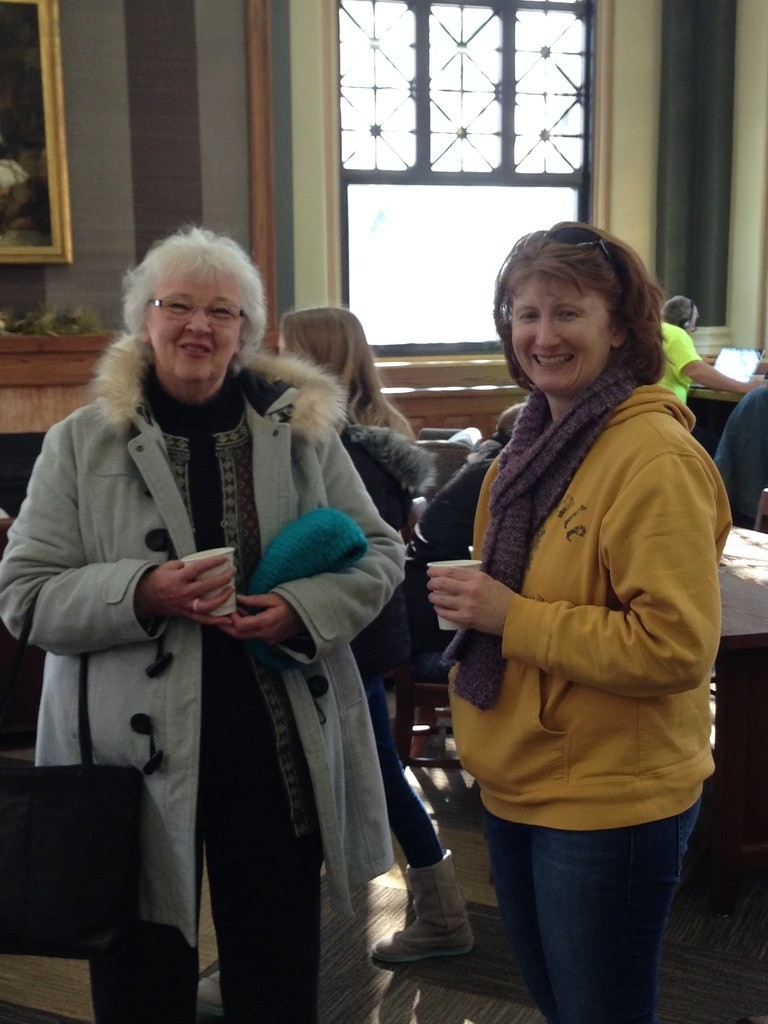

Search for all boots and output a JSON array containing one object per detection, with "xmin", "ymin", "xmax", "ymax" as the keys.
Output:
[{"xmin": 372, "ymin": 851, "xmax": 473, "ymax": 961}]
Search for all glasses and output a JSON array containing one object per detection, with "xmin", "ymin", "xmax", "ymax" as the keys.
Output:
[
  {"xmin": 147, "ymin": 295, "xmax": 245, "ymax": 326},
  {"xmin": 511, "ymin": 227, "xmax": 620, "ymax": 280}
]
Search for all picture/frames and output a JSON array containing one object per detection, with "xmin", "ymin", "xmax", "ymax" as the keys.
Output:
[{"xmin": 0, "ymin": 2, "xmax": 73, "ymax": 267}]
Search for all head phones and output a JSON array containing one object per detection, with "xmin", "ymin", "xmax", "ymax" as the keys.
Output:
[{"xmin": 679, "ymin": 300, "xmax": 694, "ymax": 328}]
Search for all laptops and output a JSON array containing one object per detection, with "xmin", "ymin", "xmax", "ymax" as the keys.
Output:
[{"xmin": 690, "ymin": 347, "xmax": 766, "ymax": 389}]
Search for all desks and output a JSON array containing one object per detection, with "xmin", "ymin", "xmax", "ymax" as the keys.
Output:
[
  {"xmin": 717, "ymin": 523, "xmax": 768, "ymax": 649},
  {"xmin": 684, "ymin": 378, "xmax": 746, "ymax": 405}
]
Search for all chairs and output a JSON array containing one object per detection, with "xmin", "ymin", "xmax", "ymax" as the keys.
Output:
[{"xmin": 374, "ymin": 424, "xmax": 487, "ymax": 777}]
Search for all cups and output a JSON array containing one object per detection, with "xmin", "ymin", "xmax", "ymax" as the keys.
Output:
[
  {"xmin": 181, "ymin": 546, "xmax": 236, "ymax": 617},
  {"xmin": 427, "ymin": 560, "xmax": 482, "ymax": 630}
]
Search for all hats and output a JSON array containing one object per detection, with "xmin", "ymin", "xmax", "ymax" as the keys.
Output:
[{"xmin": 249, "ymin": 509, "xmax": 368, "ymax": 614}]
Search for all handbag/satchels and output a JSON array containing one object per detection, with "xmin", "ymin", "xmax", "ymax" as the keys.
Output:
[{"xmin": 0, "ymin": 582, "xmax": 140, "ymax": 959}]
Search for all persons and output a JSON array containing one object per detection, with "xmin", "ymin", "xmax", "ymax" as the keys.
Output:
[
  {"xmin": 711, "ymin": 367, "xmax": 768, "ymax": 532},
  {"xmin": 0, "ymin": 229, "xmax": 411, "ymax": 1024},
  {"xmin": 423, "ymin": 215, "xmax": 736, "ymax": 1023},
  {"xmin": 655, "ymin": 287, "xmax": 765, "ymax": 426},
  {"xmin": 277, "ymin": 299, "xmax": 525, "ymax": 962}
]
